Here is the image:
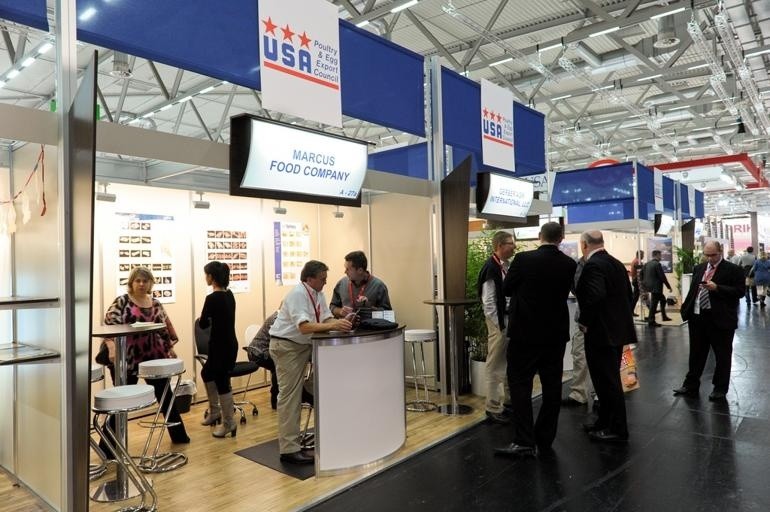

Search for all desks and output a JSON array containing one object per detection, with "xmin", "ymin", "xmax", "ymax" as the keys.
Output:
[{"xmin": 92, "ymin": 324, "xmax": 167, "ymax": 502}]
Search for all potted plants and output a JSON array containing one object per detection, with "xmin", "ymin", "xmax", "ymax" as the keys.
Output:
[
  {"xmin": 467, "ymin": 228, "xmax": 537, "ymax": 397},
  {"xmin": 673, "ymin": 262, "xmax": 682, "ymax": 310}
]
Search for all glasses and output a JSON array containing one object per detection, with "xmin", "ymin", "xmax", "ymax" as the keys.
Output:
[
  {"xmin": 703, "ymin": 251, "xmax": 719, "ymax": 257},
  {"xmin": 501, "ymin": 240, "xmax": 514, "ymax": 245}
]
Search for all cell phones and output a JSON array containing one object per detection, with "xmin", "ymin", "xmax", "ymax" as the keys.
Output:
[{"xmin": 700, "ymin": 281, "xmax": 707, "ymax": 286}]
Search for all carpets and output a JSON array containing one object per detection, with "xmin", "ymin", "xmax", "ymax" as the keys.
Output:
[{"xmin": 234, "ymin": 425, "xmax": 314, "ymax": 480}]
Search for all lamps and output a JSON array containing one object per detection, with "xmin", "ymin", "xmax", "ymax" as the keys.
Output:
[
  {"xmin": 192, "ymin": 190, "xmax": 210, "ymax": 209},
  {"xmin": 96, "ymin": 180, "xmax": 116, "ymax": 202},
  {"xmin": 274, "ymin": 200, "xmax": 286, "ymax": 214},
  {"xmin": 334, "ymin": 206, "xmax": 345, "ymax": 218}
]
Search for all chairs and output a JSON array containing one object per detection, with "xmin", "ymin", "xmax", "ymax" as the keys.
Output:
[{"xmin": 194, "ymin": 317, "xmax": 258, "ymax": 425}]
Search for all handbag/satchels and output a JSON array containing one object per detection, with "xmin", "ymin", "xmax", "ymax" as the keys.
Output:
[
  {"xmin": 95, "ymin": 294, "xmax": 129, "ymax": 364},
  {"xmin": 745, "ymin": 264, "xmax": 756, "ymax": 287}
]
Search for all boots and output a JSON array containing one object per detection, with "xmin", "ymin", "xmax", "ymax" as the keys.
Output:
[
  {"xmin": 212, "ymin": 392, "xmax": 237, "ymax": 437},
  {"xmin": 201, "ymin": 381, "xmax": 222, "ymax": 425}
]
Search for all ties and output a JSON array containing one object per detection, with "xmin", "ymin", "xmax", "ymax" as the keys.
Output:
[{"xmin": 699, "ymin": 265, "xmax": 714, "ymax": 309}]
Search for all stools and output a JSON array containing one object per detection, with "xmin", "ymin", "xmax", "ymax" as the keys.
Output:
[
  {"xmin": 300, "ymin": 403, "xmax": 315, "ymax": 450},
  {"xmin": 243, "ymin": 345, "xmax": 266, "ymax": 402},
  {"xmin": 137, "ymin": 359, "xmax": 187, "ymax": 473},
  {"xmin": 404, "ymin": 329, "xmax": 438, "ymax": 413},
  {"xmin": 89, "ymin": 366, "xmax": 107, "ymax": 481},
  {"xmin": 92, "ymin": 384, "xmax": 159, "ymax": 512}
]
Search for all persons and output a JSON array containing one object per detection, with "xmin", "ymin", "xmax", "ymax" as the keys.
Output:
[
  {"xmin": 738, "ymin": 247, "xmax": 760, "ymax": 303},
  {"xmin": 561, "ymin": 257, "xmax": 601, "ymax": 411},
  {"xmin": 330, "ymin": 251, "xmax": 392, "ymax": 321},
  {"xmin": 575, "ymin": 230, "xmax": 637, "ymax": 442},
  {"xmin": 631, "ymin": 250, "xmax": 661, "ymax": 317},
  {"xmin": 247, "ymin": 300, "xmax": 314, "ymax": 408},
  {"xmin": 195, "ymin": 261, "xmax": 238, "ymax": 439},
  {"xmin": 753, "ymin": 251, "xmax": 770, "ymax": 308},
  {"xmin": 640, "ymin": 250, "xmax": 672, "ymax": 328},
  {"xmin": 672, "ymin": 241, "xmax": 745, "ymax": 400},
  {"xmin": 96, "ymin": 267, "xmax": 190, "ymax": 460},
  {"xmin": 479, "ymin": 231, "xmax": 516, "ymax": 425},
  {"xmin": 269, "ymin": 260, "xmax": 360, "ymax": 463},
  {"xmin": 725, "ymin": 249, "xmax": 740, "ymax": 265},
  {"xmin": 494, "ymin": 222, "xmax": 577, "ymax": 457}
]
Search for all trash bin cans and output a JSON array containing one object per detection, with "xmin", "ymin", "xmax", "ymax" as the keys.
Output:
[{"xmin": 170, "ymin": 379, "xmax": 192, "ymax": 414}]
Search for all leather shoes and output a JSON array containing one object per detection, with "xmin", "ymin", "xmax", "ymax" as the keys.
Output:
[
  {"xmin": 709, "ymin": 392, "xmax": 725, "ymax": 400},
  {"xmin": 593, "ymin": 398, "xmax": 598, "ymax": 411},
  {"xmin": 486, "ymin": 409, "xmax": 510, "ymax": 423},
  {"xmin": 106, "ymin": 454, "xmax": 115, "ymax": 460},
  {"xmin": 663, "ymin": 317, "xmax": 671, "ymax": 320},
  {"xmin": 172, "ymin": 438, "xmax": 189, "ymax": 444},
  {"xmin": 633, "ymin": 313, "xmax": 638, "ymax": 316},
  {"xmin": 589, "ymin": 429, "xmax": 628, "ymax": 441},
  {"xmin": 560, "ymin": 398, "xmax": 580, "ymax": 408},
  {"xmin": 673, "ymin": 388, "xmax": 701, "ymax": 402},
  {"xmin": 538, "ymin": 446, "xmax": 557, "ymax": 465},
  {"xmin": 655, "ymin": 310, "xmax": 660, "ymax": 313},
  {"xmin": 761, "ymin": 302, "xmax": 766, "ymax": 306},
  {"xmin": 280, "ymin": 451, "xmax": 315, "ymax": 465},
  {"xmin": 580, "ymin": 422, "xmax": 599, "ymax": 431},
  {"xmin": 753, "ymin": 298, "xmax": 760, "ymax": 303},
  {"xmin": 649, "ymin": 322, "xmax": 661, "ymax": 327},
  {"xmin": 492, "ymin": 442, "xmax": 535, "ymax": 465},
  {"xmin": 747, "ymin": 302, "xmax": 750, "ymax": 305}
]
